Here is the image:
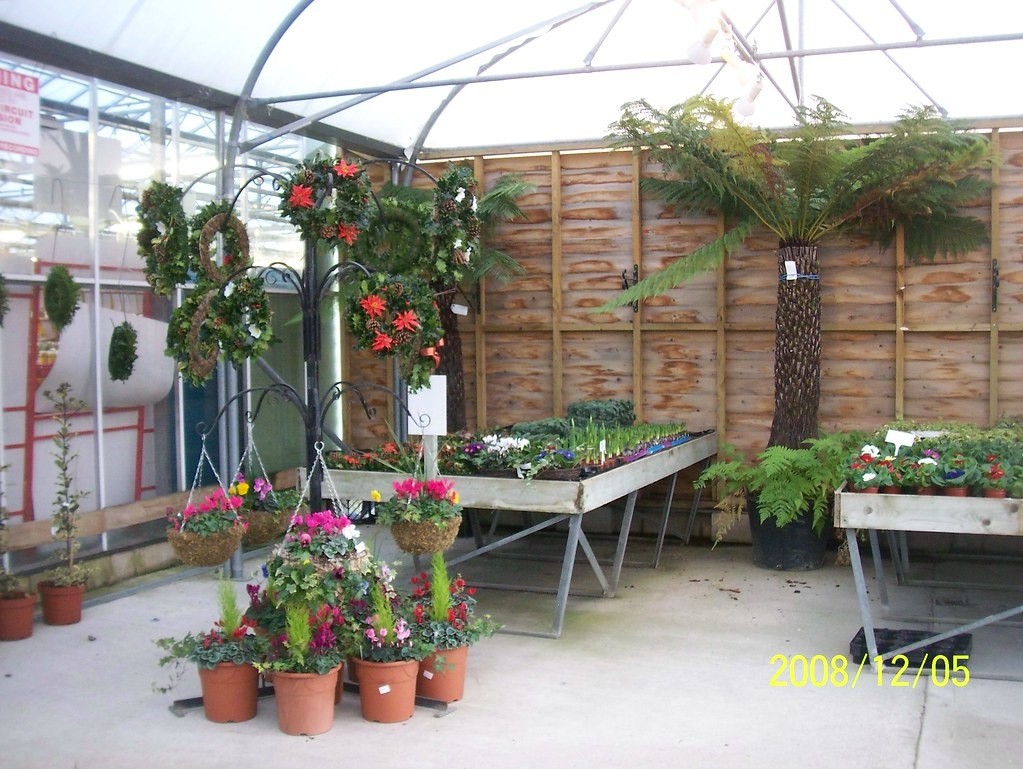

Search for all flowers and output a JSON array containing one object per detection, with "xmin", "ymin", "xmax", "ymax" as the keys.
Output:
[
  {"xmin": 844, "ymin": 415, "xmax": 1023, "ymax": 497},
  {"xmin": 129, "ymin": 147, "xmax": 487, "ymax": 395},
  {"xmin": 371, "ymin": 476, "xmax": 464, "ymax": 536},
  {"xmin": 41, "ymin": 381, "xmax": 102, "ymax": 584},
  {"xmin": 162, "ymin": 488, "xmax": 250, "ymax": 536},
  {"xmin": 227, "ymin": 473, "xmax": 282, "ymax": 513},
  {"xmin": 316, "ymin": 427, "xmax": 581, "ymax": 478},
  {"xmin": 152, "ymin": 512, "xmax": 505, "ymax": 693}
]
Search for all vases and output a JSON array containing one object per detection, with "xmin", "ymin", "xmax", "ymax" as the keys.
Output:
[
  {"xmin": 535, "ymin": 462, "xmax": 581, "ymax": 481},
  {"xmin": 859, "ymin": 484, "xmax": 879, "ymax": 494},
  {"xmin": 37, "ymin": 579, "xmax": 83, "ymax": 627},
  {"xmin": 475, "ymin": 467, "xmax": 520, "ymax": 480},
  {"xmin": 913, "ymin": 483, "xmax": 938, "ymax": 496},
  {"xmin": 942, "ymin": 486, "xmax": 970, "ymax": 497},
  {"xmin": 883, "ymin": 483, "xmax": 905, "ymax": 494},
  {"xmin": 0, "ymin": 463, "xmax": 36, "ymax": 641},
  {"xmin": 847, "ymin": 481, "xmax": 862, "ymax": 494},
  {"xmin": 980, "ymin": 486, "xmax": 1008, "ymax": 498},
  {"xmin": 194, "ymin": 621, "xmax": 469, "ymax": 736}
]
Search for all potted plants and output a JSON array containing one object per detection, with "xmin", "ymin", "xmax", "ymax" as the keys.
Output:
[
  {"xmin": 585, "ymin": 90, "xmax": 1003, "ymax": 572},
  {"xmin": 561, "ymin": 417, "xmax": 689, "ymax": 476}
]
